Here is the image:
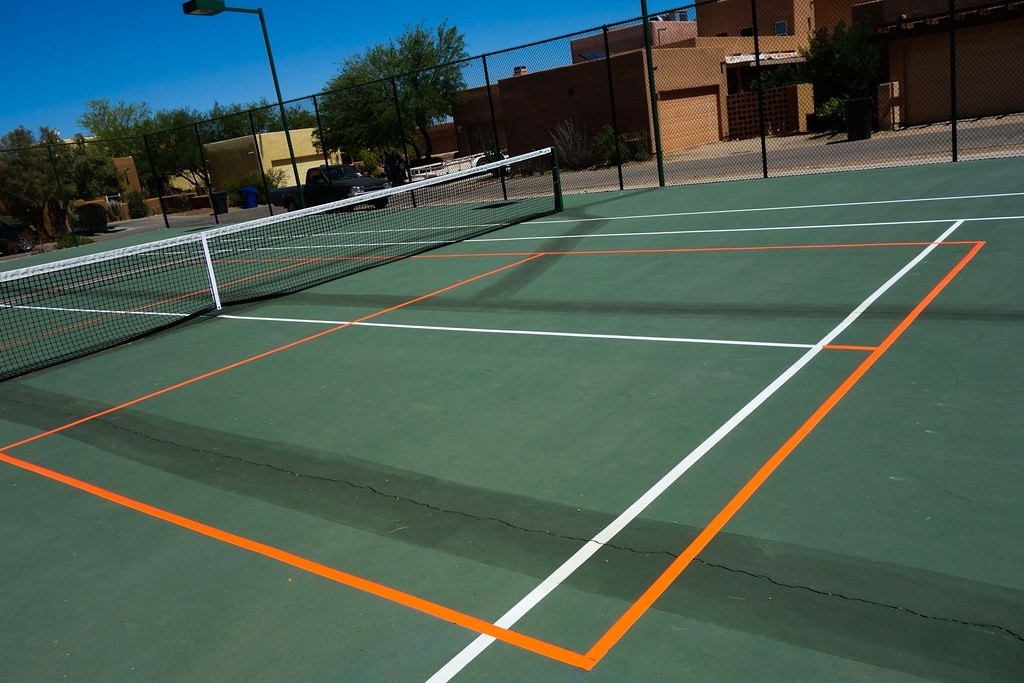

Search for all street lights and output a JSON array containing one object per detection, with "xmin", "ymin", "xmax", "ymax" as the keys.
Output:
[{"xmin": 181, "ymin": 0, "xmax": 305, "ymax": 208}]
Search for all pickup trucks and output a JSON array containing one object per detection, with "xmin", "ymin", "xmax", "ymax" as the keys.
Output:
[{"xmin": 271, "ymin": 165, "xmax": 391, "ymax": 211}]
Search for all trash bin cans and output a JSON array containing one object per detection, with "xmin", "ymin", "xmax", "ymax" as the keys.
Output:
[
  {"xmin": 238, "ymin": 187, "xmax": 257, "ymax": 208},
  {"xmin": 209, "ymin": 191, "xmax": 229, "ymax": 215}
]
